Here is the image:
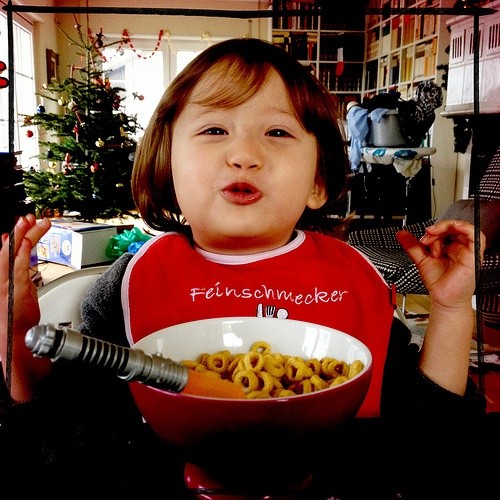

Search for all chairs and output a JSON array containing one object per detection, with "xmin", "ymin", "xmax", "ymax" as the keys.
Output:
[
  {"xmin": 346, "ymin": 147, "xmax": 500, "ymax": 314},
  {"xmin": 33, "ymin": 264, "xmax": 413, "ymax": 330}
]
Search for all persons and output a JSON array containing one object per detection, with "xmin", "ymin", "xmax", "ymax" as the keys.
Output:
[{"xmin": 0, "ymin": 39, "xmax": 485, "ymax": 446}]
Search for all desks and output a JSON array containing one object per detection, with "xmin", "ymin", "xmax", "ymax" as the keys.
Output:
[{"xmin": 344, "ymin": 145, "xmax": 437, "ymax": 227}]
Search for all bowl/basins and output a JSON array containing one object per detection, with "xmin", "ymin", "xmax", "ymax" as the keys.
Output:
[{"xmin": 120, "ymin": 317, "xmax": 376, "ymax": 493}]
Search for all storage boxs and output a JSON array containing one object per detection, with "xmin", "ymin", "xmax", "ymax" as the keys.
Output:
[
  {"xmin": 369, "ymin": 111, "xmax": 411, "ymax": 147},
  {"xmin": 31, "ymin": 217, "xmax": 134, "ymax": 272}
]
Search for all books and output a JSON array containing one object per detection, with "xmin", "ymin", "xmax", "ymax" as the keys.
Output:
[{"xmin": 273, "ymin": 1, "xmax": 439, "ymax": 120}]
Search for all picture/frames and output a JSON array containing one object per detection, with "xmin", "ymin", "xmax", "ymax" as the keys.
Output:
[{"xmin": 46, "ymin": 48, "xmax": 61, "ymax": 85}]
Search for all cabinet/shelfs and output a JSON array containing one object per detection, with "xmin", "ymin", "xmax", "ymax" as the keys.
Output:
[
  {"xmin": 268, "ymin": 0, "xmax": 456, "ymax": 123},
  {"xmin": 439, "ymin": 0, "xmax": 500, "ymax": 119}
]
